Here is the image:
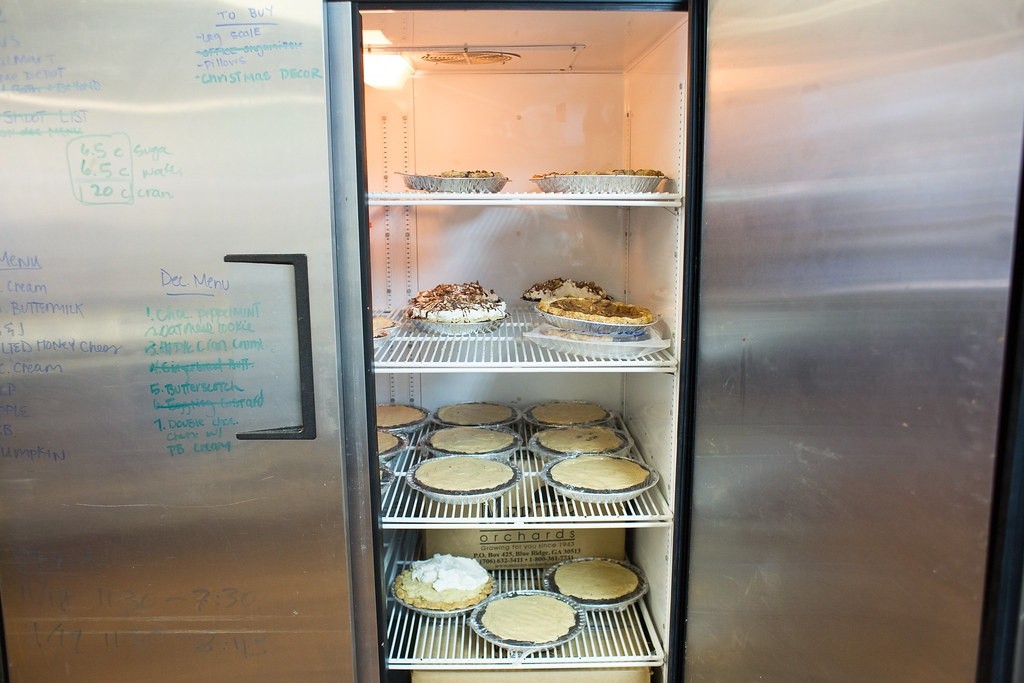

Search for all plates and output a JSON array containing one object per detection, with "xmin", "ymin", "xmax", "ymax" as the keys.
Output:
[
  {"xmin": 522, "ymin": 403, "xmax": 613, "ymax": 428},
  {"xmin": 522, "ymin": 325, "xmax": 671, "ymax": 359},
  {"xmin": 379, "ymin": 431, "xmax": 408, "ymax": 460},
  {"xmin": 541, "ymin": 455, "xmax": 660, "ymax": 503},
  {"xmin": 419, "ymin": 426, "xmax": 523, "ymax": 461},
  {"xmin": 391, "ymin": 579, "xmax": 498, "ymax": 617},
  {"xmin": 543, "ymin": 558, "xmax": 650, "ymax": 612},
  {"xmin": 380, "ymin": 462, "xmax": 395, "ymax": 494},
  {"xmin": 529, "ymin": 175, "xmax": 668, "ymax": 193},
  {"xmin": 519, "ymin": 297, "xmax": 538, "ymax": 309},
  {"xmin": 406, "ymin": 457, "xmax": 523, "ymax": 505},
  {"xmin": 469, "ymin": 590, "xmax": 588, "ymax": 651},
  {"xmin": 527, "ymin": 429, "xmax": 634, "ymax": 459},
  {"xmin": 408, "ymin": 313, "xmax": 512, "ymax": 336},
  {"xmin": 533, "ymin": 304, "xmax": 660, "ymax": 338},
  {"xmin": 430, "ymin": 402, "xmax": 522, "ymax": 427},
  {"xmin": 394, "ymin": 172, "xmax": 513, "ymax": 193},
  {"xmin": 376, "ymin": 403, "xmax": 432, "ymax": 432}
]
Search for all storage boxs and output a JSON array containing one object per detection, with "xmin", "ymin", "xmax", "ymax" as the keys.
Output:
[
  {"xmin": 412, "ymin": 580, "xmax": 651, "ymax": 683},
  {"xmin": 422, "ymin": 460, "xmax": 626, "ymax": 569}
]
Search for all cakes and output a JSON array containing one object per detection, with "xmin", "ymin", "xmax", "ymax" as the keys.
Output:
[
  {"xmin": 520, "ymin": 277, "xmax": 607, "ymax": 301},
  {"xmin": 394, "ymin": 554, "xmax": 493, "ymax": 611},
  {"xmin": 408, "ymin": 280, "xmax": 506, "ymax": 325}
]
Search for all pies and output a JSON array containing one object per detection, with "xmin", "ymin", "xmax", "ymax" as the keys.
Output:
[
  {"xmin": 531, "ymin": 168, "xmax": 663, "ymax": 178},
  {"xmin": 475, "ymin": 594, "xmax": 581, "ymax": 646},
  {"xmin": 537, "ymin": 298, "xmax": 654, "ymax": 325},
  {"xmin": 373, "ymin": 402, "xmax": 652, "ymax": 492},
  {"xmin": 548, "ymin": 559, "xmax": 644, "ymax": 604},
  {"xmin": 419, "ymin": 168, "xmax": 503, "ymax": 178}
]
